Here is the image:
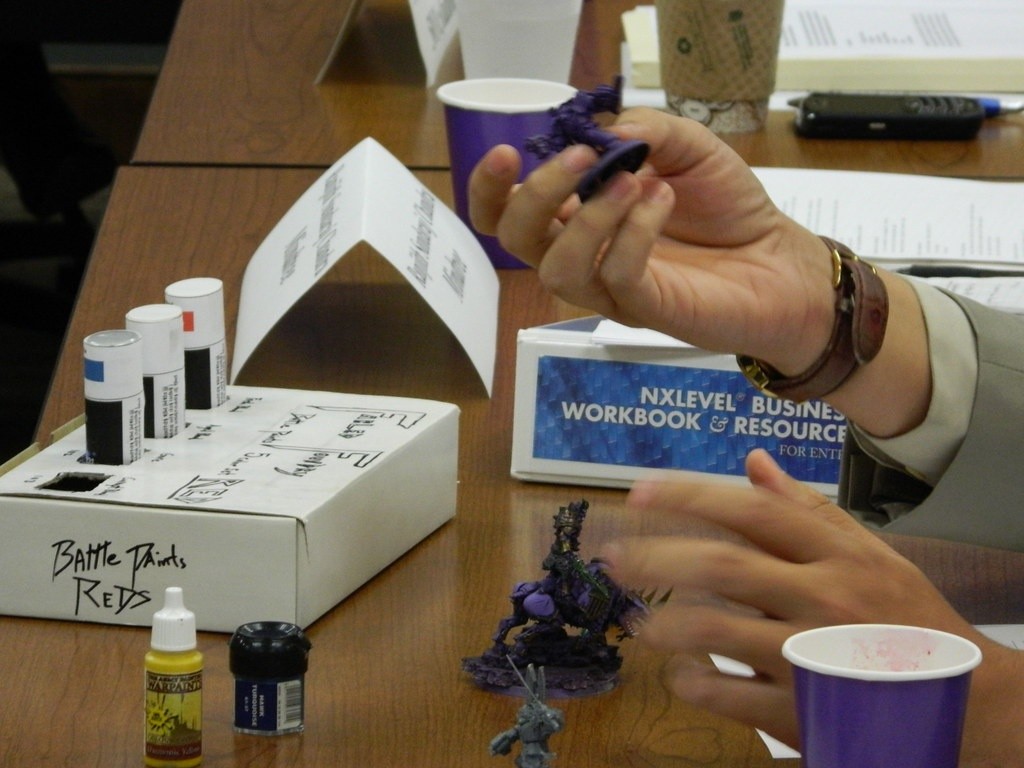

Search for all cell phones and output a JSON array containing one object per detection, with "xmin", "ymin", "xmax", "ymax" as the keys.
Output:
[{"xmin": 783, "ymin": 90, "xmax": 988, "ymax": 143}]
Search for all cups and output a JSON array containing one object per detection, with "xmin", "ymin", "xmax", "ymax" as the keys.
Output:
[
  {"xmin": 436, "ymin": 78, "xmax": 580, "ymax": 269},
  {"xmin": 781, "ymin": 623, "xmax": 983, "ymax": 768},
  {"xmin": 652, "ymin": 0, "xmax": 785, "ymax": 134}
]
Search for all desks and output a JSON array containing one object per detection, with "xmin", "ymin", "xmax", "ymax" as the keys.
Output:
[
  {"xmin": 0, "ymin": 162, "xmax": 1024, "ymax": 768},
  {"xmin": 128, "ymin": 2, "xmax": 1024, "ymax": 186}
]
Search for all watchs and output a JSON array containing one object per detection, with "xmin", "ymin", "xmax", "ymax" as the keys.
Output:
[{"xmin": 735, "ymin": 235, "xmax": 889, "ymax": 403}]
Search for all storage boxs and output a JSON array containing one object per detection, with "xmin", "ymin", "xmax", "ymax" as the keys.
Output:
[
  {"xmin": 1, "ymin": 388, "xmax": 460, "ymax": 636},
  {"xmin": 511, "ymin": 315, "xmax": 847, "ymax": 500}
]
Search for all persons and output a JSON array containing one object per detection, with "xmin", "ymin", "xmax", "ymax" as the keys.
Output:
[
  {"xmin": 537, "ymin": 497, "xmax": 609, "ymax": 636},
  {"xmin": 484, "ymin": 662, "xmax": 564, "ymax": 768},
  {"xmin": 465, "ymin": 106, "xmax": 1024, "ymax": 768}
]
